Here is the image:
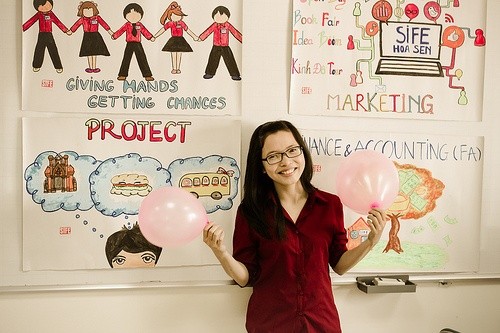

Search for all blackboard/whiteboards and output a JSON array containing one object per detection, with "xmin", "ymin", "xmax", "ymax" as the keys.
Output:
[
  {"xmin": 373, "ymin": 278, "xmax": 405, "ymax": 286},
  {"xmin": 1, "ymin": 1, "xmax": 500, "ymax": 292}
]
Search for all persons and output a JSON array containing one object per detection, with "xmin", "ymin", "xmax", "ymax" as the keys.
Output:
[{"xmin": 203, "ymin": 121, "xmax": 386, "ymax": 333}]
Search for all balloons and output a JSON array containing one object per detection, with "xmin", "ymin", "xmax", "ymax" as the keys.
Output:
[
  {"xmin": 138, "ymin": 186, "xmax": 208, "ymax": 248},
  {"xmin": 337, "ymin": 150, "xmax": 399, "ymax": 215}
]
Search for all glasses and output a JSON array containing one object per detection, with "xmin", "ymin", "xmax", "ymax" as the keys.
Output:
[{"xmin": 262, "ymin": 144, "xmax": 303, "ymax": 165}]
